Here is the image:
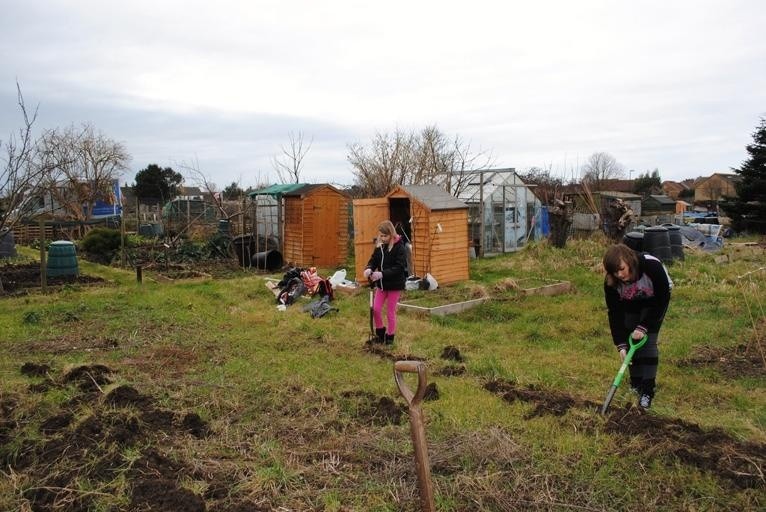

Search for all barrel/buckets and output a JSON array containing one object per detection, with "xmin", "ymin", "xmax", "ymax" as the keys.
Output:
[
  {"xmin": 252, "ymin": 248, "xmax": 284, "ymax": 273},
  {"xmin": 661, "ymin": 223, "xmax": 686, "ymax": 262},
  {"xmin": 623, "ymin": 230, "xmax": 644, "ymax": 251},
  {"xmin": 642, "ymin": 224, "xmax": 674, "ymax": 266},
  {"xmin": 234, "ymin": 239, "xmax": 255, "ymax": 267}
]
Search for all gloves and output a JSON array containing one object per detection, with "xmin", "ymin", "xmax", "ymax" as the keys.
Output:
[{"xmin": 364, "ymin": 268, "xmax": 383, "ymax": 283}]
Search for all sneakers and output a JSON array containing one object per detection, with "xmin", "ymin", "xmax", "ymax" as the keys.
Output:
[{"xmin": 628, "ymin": 386, "xmax": 651, "ymax": 408}]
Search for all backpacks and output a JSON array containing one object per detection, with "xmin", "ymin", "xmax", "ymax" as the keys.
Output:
[{"xmin": 314, "ymin": 280, "xmax": 333, "ymax": 301}]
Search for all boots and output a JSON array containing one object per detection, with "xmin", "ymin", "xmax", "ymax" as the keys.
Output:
[{"xmin": 371, "ymin": 326, "xmax": 394, "ymax": 345}]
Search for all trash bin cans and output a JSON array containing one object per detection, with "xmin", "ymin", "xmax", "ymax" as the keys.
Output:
[{"xmin": 233, "ymin": 235, "xmax": 283, "ymax": 271}]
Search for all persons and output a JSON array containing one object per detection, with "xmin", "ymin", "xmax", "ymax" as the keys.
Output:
[
  {"xmin": 397, "ymin": 206, "xmax": 412, "ymax": 277},
  {"xmin": 603, "ymin": 242, "xmax": 675, "ymax": 410},
  {"xmin": 363, "ymin": 219, "xmax": 409, "ymax": 347}
]
